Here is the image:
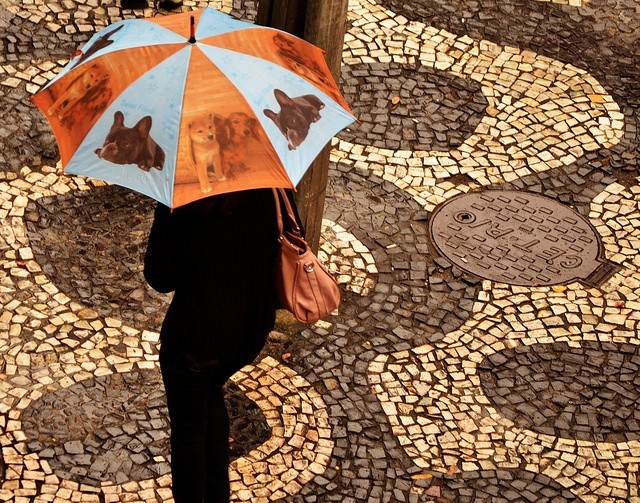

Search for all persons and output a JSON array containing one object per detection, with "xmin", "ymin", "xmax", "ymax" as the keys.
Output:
[{"xmin": 144, "ymin": 188, "xmax": 305, "ymax": 502}]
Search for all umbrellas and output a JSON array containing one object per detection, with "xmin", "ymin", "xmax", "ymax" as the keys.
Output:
[{"xmin": 27, "ymin": 4, "xmax": 361, "ymax": 214}]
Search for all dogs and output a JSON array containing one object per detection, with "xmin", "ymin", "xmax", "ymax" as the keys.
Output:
[
  {"xmin": 95, "ymin": 111, "xmax": 165, "ymax": 172},
  {"xmin": 185, "ymin": 112, "xmax": 226, "ymax": 194},
  {"xmin": 276, "ymin": 48, "xmax": 342, "ymax": 99},
  {"xmin": 273, "ymin": 33, "xmax": 326, "ymax": 76},
  {"xmin": 263, "ymin": 88, "xmax": 325, "ymax": 152},
  {"xmin": 69, "ymin": 23, "xmax": 124, "ymax": 70},
  {"xmin": 214, "ymin": 112, "xmax": 263, "ymax": 181},
  {"xmin": 47, "ymin": 62, "xmax": 112, "ymax": 136}
]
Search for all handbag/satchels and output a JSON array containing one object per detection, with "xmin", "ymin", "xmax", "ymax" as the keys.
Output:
[{"xmin": 272, "ymin": 188, "xmax": 344, "ymax": 326}]
